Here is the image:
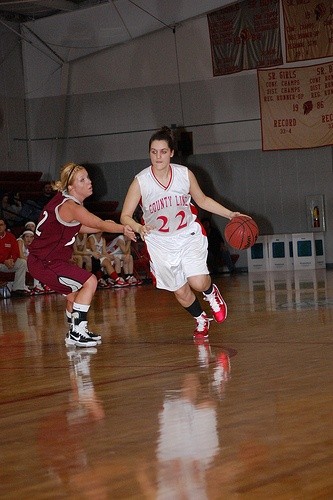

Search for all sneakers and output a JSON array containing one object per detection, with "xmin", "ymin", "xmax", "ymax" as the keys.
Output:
[
  {"xmin": 64, "ymin": 321, "xmax": 102, "ymax": 347},
  {"xmin": 10, "ymin": 285, "xmax": 57, "ymax": 297},
  {"xmin": 96, "ymin": 276, "xmax": 143, "ymax": 288},
  {"xmin": 192, "ymin": 311, "xmax": 210, "ymax": 339},
  {"xmin": 202, "ymin": 284, "xmax": 227, "ymax": 323}
]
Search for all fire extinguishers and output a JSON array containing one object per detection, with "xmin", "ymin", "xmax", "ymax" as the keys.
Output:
[{"xmin": 313, "ymin": 206, "xmax": 320, "ymax": 227}]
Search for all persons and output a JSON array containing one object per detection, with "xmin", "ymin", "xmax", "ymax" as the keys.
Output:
[
  {"xmin": 0, "ymin": 219, "xmax": 28, "ymax": 296},
  {"xmin": 0, "ymin": 191, "xmax": 41, "ymax": 226},
  {"xmin": 35, "ymin": 348, "xmax": 156, "ymax": 500},
  {"xmin": 108, "ymin": 229, "xmax": 142, "ymax": 286},
  {"xmin": 72, "ymin": 231, "xmax": 114, "ymax": 288},
  {"xmin": 16, "ymin": 231, "xmax": 54, "ymax": 294},
  {"xmin": 136, "ymin": 341, "xmax": 247, "ymax": 500},
  {"xmin": 120, "ymin": 126, "xmax": 252, "ymax": 338},
  {"xmin": 27, "ymin": 163, "xmax": 137, "ymax": 347},
  {"xmin": 41, "ymin": 184, "xmax": 56, "ymax": 207},
  {"xmin": 25, "ymin": 222, "xmax": 36, "ymax": 232},
  {"xmin": 87, "ymin": 231, "xmax": 130, "ymax": 287}
]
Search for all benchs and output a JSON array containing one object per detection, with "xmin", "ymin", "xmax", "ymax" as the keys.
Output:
[{"xmin": 0, "ymin": 171, "xmax": 122, "ymax": 283}]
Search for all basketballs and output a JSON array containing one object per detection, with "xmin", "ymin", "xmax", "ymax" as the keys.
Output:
[{"xmin": 225, "ymin": 216, "xmax": 259, "ymax": 250}]
